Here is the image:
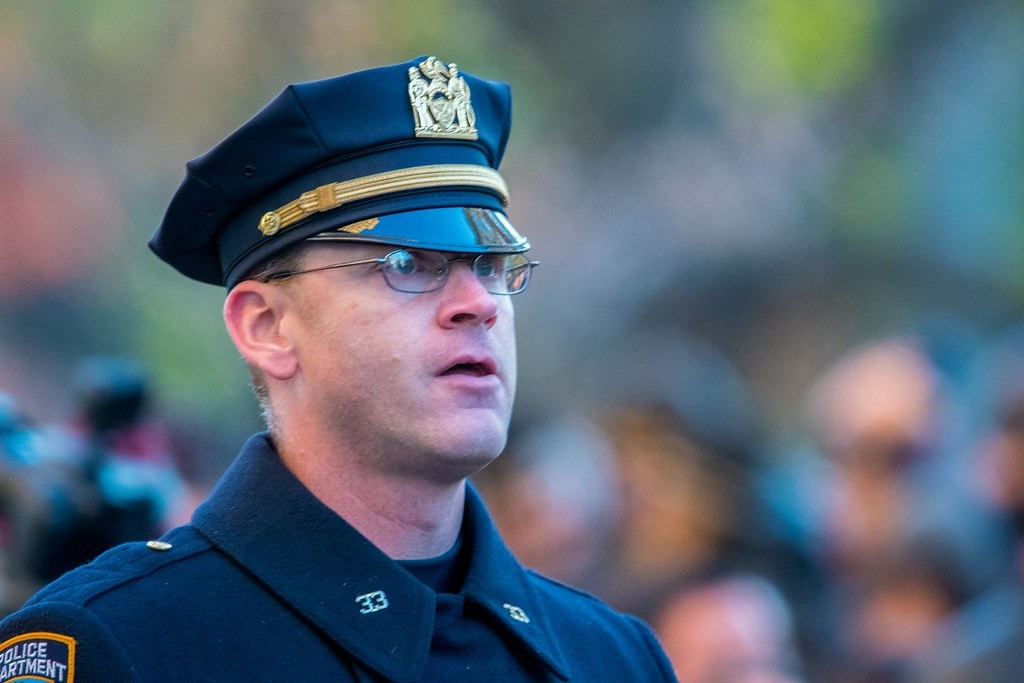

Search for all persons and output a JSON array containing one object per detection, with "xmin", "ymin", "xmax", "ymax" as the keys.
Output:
[
  {"xmin": 1, "ymin": 55, "xmax": 675, "ymax": 683},
  {"xmin": 0, "ymin": 343, "xmax": 1023, "ymax": 683}
]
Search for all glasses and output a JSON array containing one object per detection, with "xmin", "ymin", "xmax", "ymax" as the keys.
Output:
[{"xmin": 264, "ymin": 247, "xmax": 541, "ymax": 294}]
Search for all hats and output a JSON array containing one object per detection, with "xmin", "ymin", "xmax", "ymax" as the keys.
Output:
[{"xmin": 146, "ymin": 55, "xmax": 532, "ymax": 296}]
